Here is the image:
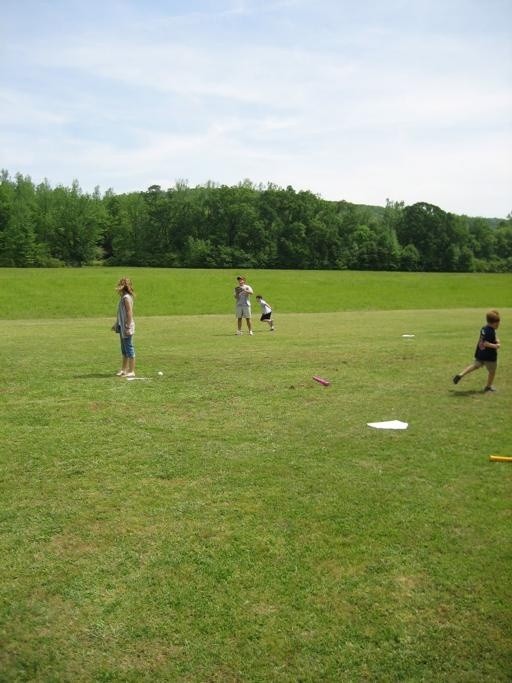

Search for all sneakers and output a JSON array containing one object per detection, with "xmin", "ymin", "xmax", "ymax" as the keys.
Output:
[
  {"xmin": 453, "ymin": 374, "xmax": 461, "ymax": 385},
  {"xmin": 269, "ymin": 320, "xmax": 273, "ymax": 327},
  {"xmin": 248, "ymin": 331, "xmax": 253, "ymax": 335},
  {"xmin": 121, "ymin": 372, "xmax": 135, "ymax": 377},
  {"xmin": 116, "ymin": 370, "xmax": 126, "ymax": 375},
  {"xmin": 484, "ymin": 386, "xmax": 497, "ymax": 392},
  {"xmin": 270, "ymin": 327, "xmax": 274, "ymax": 330},
  {"xmin": 235, "ymin": 330, "xmax": 242, "ymax": 335}
]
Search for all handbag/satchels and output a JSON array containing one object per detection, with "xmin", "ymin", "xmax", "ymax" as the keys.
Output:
[{"xmin": 115, "ymin": 324, "xmax": 121, "ymax": 333}]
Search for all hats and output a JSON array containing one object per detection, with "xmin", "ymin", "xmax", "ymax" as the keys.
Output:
[
  {"xmin": 116, "ymin": 277, "xmax": 131, "ymax": 288},
  {"xmin": 237, "ymin": 275, "xmax": 245, "ymax": 281}
]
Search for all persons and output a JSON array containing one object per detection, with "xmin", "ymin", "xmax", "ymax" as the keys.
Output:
[
  {"xmin": 450, "ymin": 307, "xmax": 503, "ymax": 392},
  {"xmin": 234, "ymin": 274, "xmax": 252, "ymax": 335},
  {"xmin": 255, "ymin": 292, "xmax": 274, "ymax": 329},
  {"xmin": 115, "ymin": 275, "xmax": 138, "ymax": 377}
]
